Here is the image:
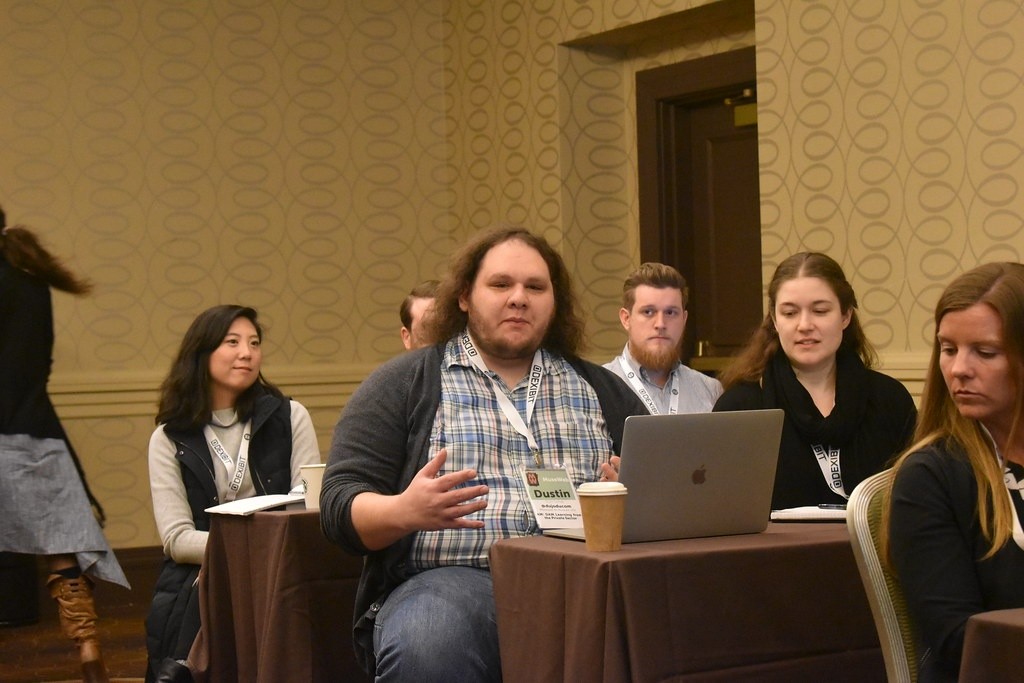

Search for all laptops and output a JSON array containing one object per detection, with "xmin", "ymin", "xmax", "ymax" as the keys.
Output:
[{"xmin": 542, "ymin": 409, "xmax": 785, "ymax": 542}]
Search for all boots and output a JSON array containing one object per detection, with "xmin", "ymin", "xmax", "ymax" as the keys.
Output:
[{"xmin": 41, "ymin": 563, "xmax": 115, "ymax": 682}]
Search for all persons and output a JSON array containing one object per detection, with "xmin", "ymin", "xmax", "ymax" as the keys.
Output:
[
  {"xmin": 142, "ymin": 304, "xmax": 321, "ymax": 683},
  {"xmin": 0, "ymin": 208, "xmax": 133, "ymax": 683},
  {"xmin": 712, "ymin": 252, "xmax": 918, "ymax": 509},
  {"xmin": 886, "ymin": 262, "xmax": 1024, "ymax": 683},
  {"xmin": 319, "ymin": 229, "xmax": 726, "ymax": 683}
]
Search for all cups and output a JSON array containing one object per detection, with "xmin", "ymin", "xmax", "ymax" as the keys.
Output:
[
  {"xmin": 577, "ymin": 481, "xmax": 628, "ymax": 552},
  {"xmin": 301, "ymin": 463, "xmax": 327, "ymax": 510}
]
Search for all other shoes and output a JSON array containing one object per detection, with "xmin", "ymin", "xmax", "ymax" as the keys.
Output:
[{"xmin": 154, "ymin": 656, "xmax": 195, "ymax": 683}]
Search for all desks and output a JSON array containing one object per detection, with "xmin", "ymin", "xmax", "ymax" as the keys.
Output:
[
  {"xmin": 492, "ymin": 519, "xmax": 888, "ymax": 683},
  {"xmin": 958, "ymin": 607, "xmax": 1024, "ymax": 683},
  {"xmin": 207, "ymin": 510, "xmax": 369, "ymax": 683}
]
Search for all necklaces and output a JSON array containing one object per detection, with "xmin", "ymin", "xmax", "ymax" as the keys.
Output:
[{"xmin": 210, "ymin": 420, "xmax": 238, "ymax": 428}]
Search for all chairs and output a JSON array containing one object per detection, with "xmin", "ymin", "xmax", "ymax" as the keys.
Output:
[{"xmin": 845, "ymin": 467, "xmax": 931, "ymax": 683}]
